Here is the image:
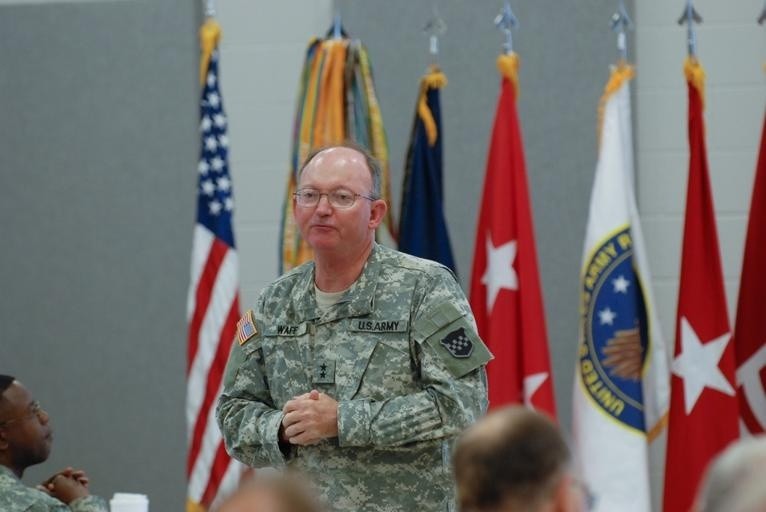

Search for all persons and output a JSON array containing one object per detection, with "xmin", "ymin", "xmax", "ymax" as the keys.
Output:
[
  {"xmin": 0, "ymin": 375, "xmax": 107, "ymax": 512},
  {"xmin": 451, "ymin": 403, "xmax": 599, "ymax": 512},
  {"xmin": 210, "ymin": 468, "xmax": 326, "ymax": 512},
  {"xmin": 213, "ymin": 141, "xmax": 495, "ymax": 512},
  {"xmin": 693, "ymin": 436, "xmax": 766, "ymax": 512}
]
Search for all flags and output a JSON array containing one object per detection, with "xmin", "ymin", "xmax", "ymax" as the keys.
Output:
[
  {"xmin": 734, "ymin": 116, "xmax": 766, "ymax": 432},
  {"xmin": 469, "ymin": 54, "xmax": 556, "ymax": 419},
  {"xmin": 280, "ymin": 40, "xmax": 399, "ymax": 277},
  {"xmin": 184, "ymin": 16, "xmax": 241, "ymax": 512},
  {"xmin": 663, "ymin": 56, "xmax": 742, "ymax": 512},
  {"xmin": 399, "ymin": 66, "xmax": 456, "ymax": 274},
  {"xmin": 570, "ymin": 59, "xmax": 671, "ymax": 512}
]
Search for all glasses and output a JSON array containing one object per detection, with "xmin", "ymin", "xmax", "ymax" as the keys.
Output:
[
  {"xmin": 1, "ymin": 400, "xmax": 41, "ymax": 429},
  {"xmin": 292, "ymin": 187, "xmax": 375, "ymax": 209}
]
